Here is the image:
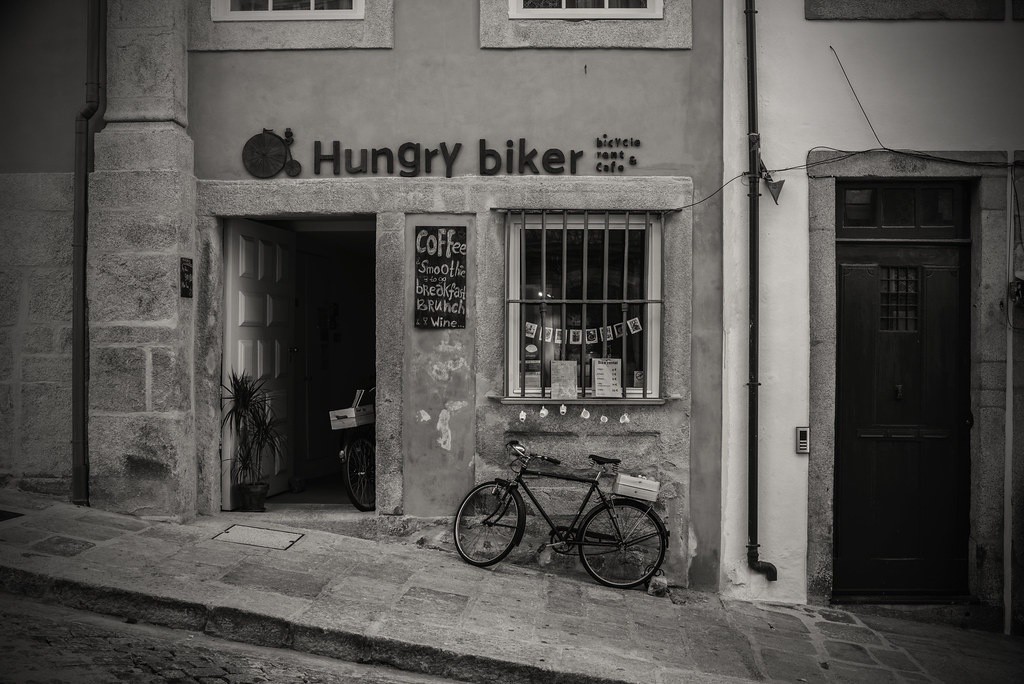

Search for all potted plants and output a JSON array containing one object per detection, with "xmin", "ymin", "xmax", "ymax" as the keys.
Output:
[{"xmin": 219, "ymin": 363, "xmax": 294, "ymax": 512}]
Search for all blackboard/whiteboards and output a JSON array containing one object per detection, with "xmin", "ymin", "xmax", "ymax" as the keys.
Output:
[{"xmin": 414, "ymin": 225, "xmax": 467, "ymax": 330}]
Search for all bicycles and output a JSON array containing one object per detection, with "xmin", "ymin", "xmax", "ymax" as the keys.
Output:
[
  {"xmin": 454, "ymin": 440, "xmax": 671, "ymax": 591},
  {"xmin": 339, "ymin": 385, "xmax": 377, "ymax": 512}
]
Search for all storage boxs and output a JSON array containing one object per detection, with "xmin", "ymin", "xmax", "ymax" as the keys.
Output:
[
  {"xmin": 612, "ymin": 473, "xmax": 661, "ymax": 503},
  {"xmin": 329, "ymin": 404, "xmax": 375, "ymax": 430}
]
